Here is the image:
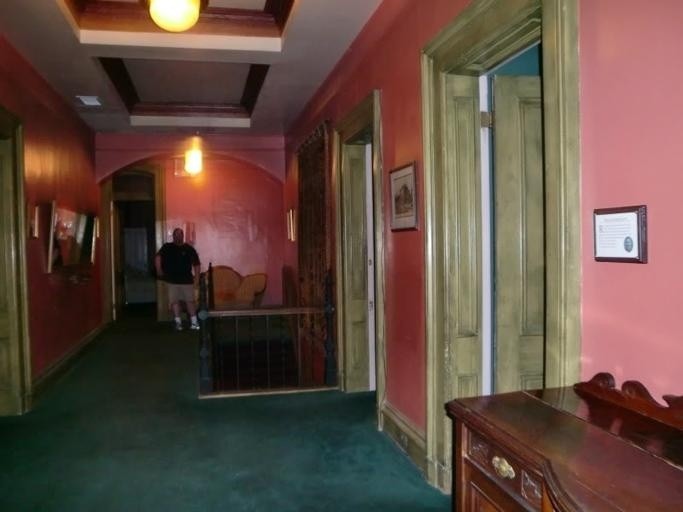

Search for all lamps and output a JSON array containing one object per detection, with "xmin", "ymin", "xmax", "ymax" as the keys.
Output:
[{"xmin": 149, "ymin": 0, "xmax": 202, "ymax": 34}]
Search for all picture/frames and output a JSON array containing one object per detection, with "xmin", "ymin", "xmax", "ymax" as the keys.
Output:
[
  {"xmin": 592, "ymin": 205, "xmax": 647, "ymax": 264},
  {"xmin": 388, "ymin": 161, "xmax": 418, "ymax": 232},
  {"xmin": 286, "ymin": 210, "xmax": 296, "ymax": 243},
  {"xmin": 97, "ymin": 219, "xmax": 103, "ymax": 240},
  {"xmin": 32, "ymin": 206, "xmax": 42, "ymax": 239}
]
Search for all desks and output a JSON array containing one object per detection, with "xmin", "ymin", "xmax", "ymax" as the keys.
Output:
[{"xmin": 194, "ymin": 304, "xmax": 339, "ymax": 400}]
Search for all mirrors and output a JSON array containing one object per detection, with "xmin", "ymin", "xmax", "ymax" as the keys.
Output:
[{"xmin": 48, "ymin": 202, "xmax": 97, "ymax": 271}]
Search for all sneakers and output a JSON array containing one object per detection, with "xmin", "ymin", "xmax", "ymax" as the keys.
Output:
[{"xmin": 175, "ymin": 322, "xmax": 200, "ymax": 333}]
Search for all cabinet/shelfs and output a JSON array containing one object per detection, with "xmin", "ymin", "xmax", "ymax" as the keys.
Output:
[{"xmin": 444, "ymin": 372, "xmax": 683, "ymax": 512}]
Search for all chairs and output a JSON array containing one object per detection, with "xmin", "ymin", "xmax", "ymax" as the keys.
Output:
[{"xmin": 157, "ymin": 266, "xmax": 267, "ymax": 323}]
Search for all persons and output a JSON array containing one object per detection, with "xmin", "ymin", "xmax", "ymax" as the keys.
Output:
[{"xmin": 154, "ymin": 227, "xmax": 202, "ymax": 331}]
